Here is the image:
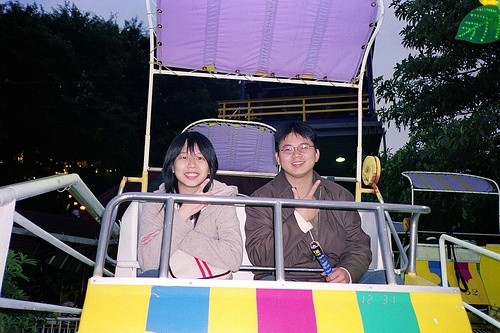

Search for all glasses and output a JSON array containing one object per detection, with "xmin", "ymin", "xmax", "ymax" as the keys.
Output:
[{"xmin": 277, "ymin": 144, "xmax": 316, "ymax": 154}]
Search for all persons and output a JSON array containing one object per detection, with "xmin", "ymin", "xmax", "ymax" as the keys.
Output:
[
  {"xmin": 138, "ymin": 130, "xmax": 243, "ymax": 280},
  {"xmin": 244, "ymin": 119, "xmax": 405, "ymax": 283}
]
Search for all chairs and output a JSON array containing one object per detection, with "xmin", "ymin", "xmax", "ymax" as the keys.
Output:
[{"xmin": 116, "ymin": 193, "xmax": 394, "ymax": 284}]
